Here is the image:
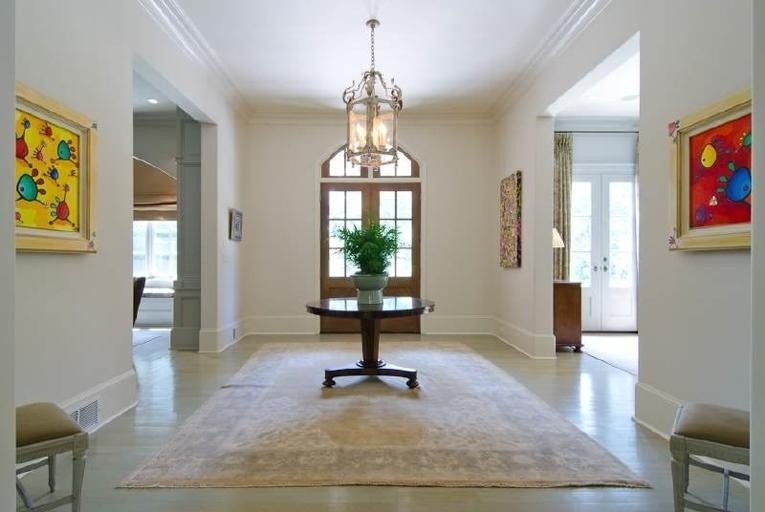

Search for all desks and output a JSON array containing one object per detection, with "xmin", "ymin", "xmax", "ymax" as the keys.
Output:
[
  {"xmin": 306, "ymin": 297, "xmax": 434, "ymax": 388},
  {"xmin": 553, "ymin": 281, "xmax": 584, "ymax": 352}
]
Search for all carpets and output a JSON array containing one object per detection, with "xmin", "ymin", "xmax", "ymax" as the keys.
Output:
[{"xmin": 114, "ymin": 343, "xmax": 653, "ymax": 488}]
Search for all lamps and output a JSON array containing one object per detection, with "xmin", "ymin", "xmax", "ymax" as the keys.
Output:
[
  {"xmin": 342, "ymin": 20, "xmax": 402, "ymax": 169},
  {"xmin": 552, "ymin": 227, "xmax": 565, "ymax": 248}
]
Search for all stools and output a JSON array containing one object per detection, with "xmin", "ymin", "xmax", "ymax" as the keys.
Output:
[
  {"xmin": 670, "ymin": 403, "xmax": 750, "ymax": 512},
  {"xmin": 15, "ymin": 401, "xmax": 89, "ymax": 512}
]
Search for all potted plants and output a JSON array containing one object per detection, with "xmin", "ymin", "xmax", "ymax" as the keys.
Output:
[{"xmin": 331, "ymin": 220, "xmax": 400, "ymax": 305}]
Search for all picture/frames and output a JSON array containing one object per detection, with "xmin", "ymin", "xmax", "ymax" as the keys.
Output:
[
  {"xmin": 14, "ymin": 82, "xmax": 97, "ymax": 254},
  {"xmin": 229, "ymin": 208, "xmax": 242, "ymax": 241},
  {"xmin": 667, "ymin": 87, "xmax": 752, "ymax": 252}
]
